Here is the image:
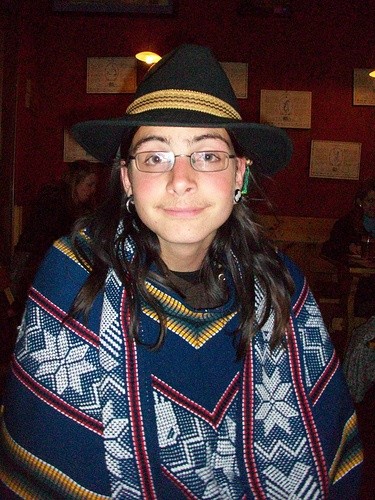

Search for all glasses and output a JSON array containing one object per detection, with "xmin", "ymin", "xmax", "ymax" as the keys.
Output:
[
  {"xmin": 126, "ymin": 151, "xmax": 238, "ymax": 173},
  {"xmin": 361, "ymin": 198, "xmax": 375, "ymax": 206}
]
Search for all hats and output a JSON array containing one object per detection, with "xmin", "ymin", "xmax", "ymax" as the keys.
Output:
[{"xmin": 70, "ymin": 44, "xmax": 292, "ymax": 175}]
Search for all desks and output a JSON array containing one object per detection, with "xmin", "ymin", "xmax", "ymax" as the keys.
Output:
[{"xmin": 319, "ymin": 251, "xmax": 375, "ymax": 352}]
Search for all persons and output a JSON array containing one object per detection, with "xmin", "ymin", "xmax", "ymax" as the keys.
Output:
[
  {"xmin": 330, "ymin": 181, "xmax": 375, "ymax": 320},
  {"xmin": 0, "ymin": 43, "xmax": 367, "ymax": 500}
]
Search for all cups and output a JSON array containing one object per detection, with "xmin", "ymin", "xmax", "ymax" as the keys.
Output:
[{"xmin": 360, "ymin": 234, "xmax": 375, "ymax": 260}]
